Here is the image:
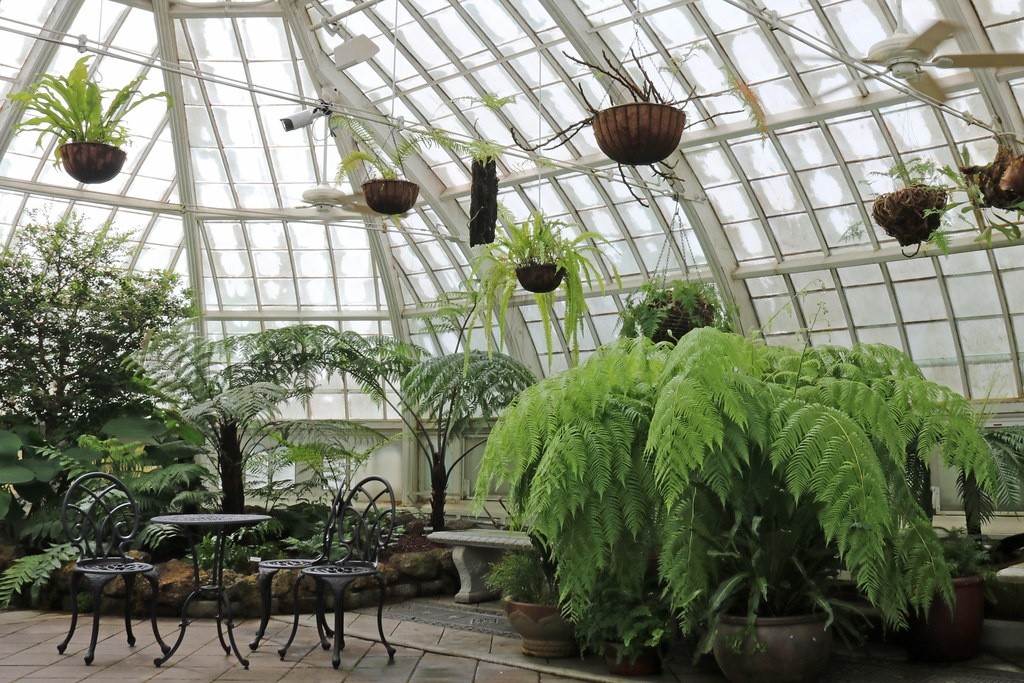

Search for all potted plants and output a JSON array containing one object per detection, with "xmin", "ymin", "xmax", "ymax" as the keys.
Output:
[
  {"xmin": 7, "ymin": 52, "xmax": 172, "ymax": 185},
  {"xmin": 485, "ymin": 333, "xmax": 1024, "ymax": 683},
  {"xmin": 327, "ymin": 111, "xmax": 457, "ymax": 214},
  {"xmin": 841, "ymin": 155, "xmax": 965, "ymax": 260},
  {"xmin": 616, "ymin": 279, "xmax": 741, "ymax": 347},
  {"xmin": 451, "ymin": 203, "xmax": 622, "ymax": 324}
]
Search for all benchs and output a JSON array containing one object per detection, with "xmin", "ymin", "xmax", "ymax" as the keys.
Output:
[{"xmin": 429, "ymin": 527, "xmax": 555, "ymax": 604}]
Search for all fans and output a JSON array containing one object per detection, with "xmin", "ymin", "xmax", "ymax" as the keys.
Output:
[
  {"xmin": 266, "ymin": 112, "xmax": 410, "ymax": 219},
  {"xmin": 774, "ymin": 0, "xmax": 1024, "ymax": 110}
]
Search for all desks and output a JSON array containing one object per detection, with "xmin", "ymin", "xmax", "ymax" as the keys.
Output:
[{"xmin": 149, "ymin": 513, "xmax": 272, "ymax": 671}]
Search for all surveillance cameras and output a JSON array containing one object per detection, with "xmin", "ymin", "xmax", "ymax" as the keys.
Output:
[{"xmin": 280, "ymin": 109, "xmax": 313, "ymax": 132}]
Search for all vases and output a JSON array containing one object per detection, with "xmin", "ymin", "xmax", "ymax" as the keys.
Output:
[{"xmin": 588, "ymin": 103, "xmax": 686, "ymax": 166}]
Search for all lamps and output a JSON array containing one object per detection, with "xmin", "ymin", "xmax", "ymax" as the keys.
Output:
[{"xmin": 325, "ymin": 16, "xmax": 382, "ymax": 73}]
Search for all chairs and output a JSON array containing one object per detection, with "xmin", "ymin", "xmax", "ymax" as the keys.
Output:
[
  {"xmin": 248, "ymin": 476, "xmax": 396, "ymax": 671},
  {"xmin": 55, "ymin": 472, "xmax": 172, "ymax": 664}
]
